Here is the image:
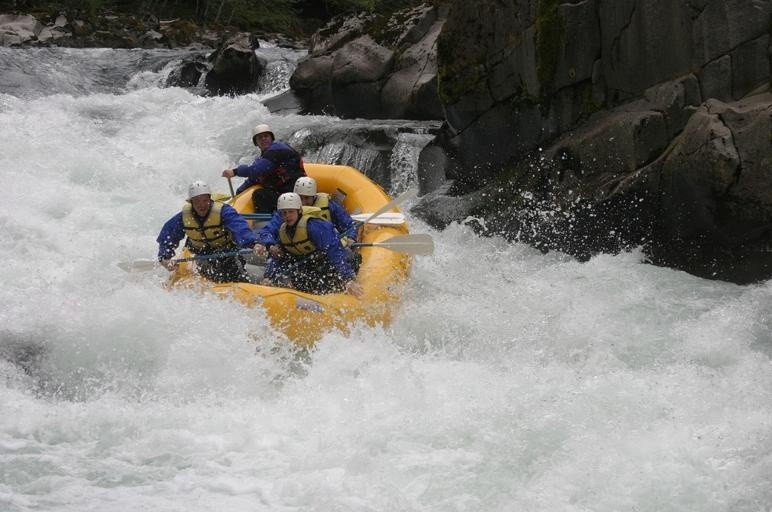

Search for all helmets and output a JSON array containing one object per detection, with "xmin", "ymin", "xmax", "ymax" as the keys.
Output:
[
  {"xmin": 294, "ymin": 177, "xmax": 318, "ymax": 196},
  {"xmin": 278, "ymin": 193, "xmax": 300, "ymax": 209},
  {"xmin": 252, "ymin": 125, "xmax": 275, "ymax": 146},
  {"xmin": 184, "ymin": 181, "xmax": 210, "ymax": 201}
]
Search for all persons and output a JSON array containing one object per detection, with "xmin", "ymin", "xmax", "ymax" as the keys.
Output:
[
  {"xmin": 259, "ymin": 192, "xmax": 366, "ymax": 299},
  {"xmin": 222, "ymin": 124, "xmax": 307, "ymax": 214},
  {"xmin": 268, "ymin": 176, "xmax": 359, "ymax": 274},
  {"xmin": 156, "ymin": 181, "xmax": 266, "ymax": 284}
]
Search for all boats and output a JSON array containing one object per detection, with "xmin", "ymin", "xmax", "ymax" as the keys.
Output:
[{"xmin": 154, "ymin": 162, "xmax": 418, "ymax": 348}]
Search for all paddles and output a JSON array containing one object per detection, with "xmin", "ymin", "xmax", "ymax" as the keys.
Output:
[
  {"xmin": 348, "ymin": 234, "xmax": 435, "ymax": 258},
  {"xmin": 239, "ymin": 186, "xmax": 419, "ymax": 284},
  {"xmin": 117, "ymin": 247, "xmax": 255, "ymax": 274}
]
